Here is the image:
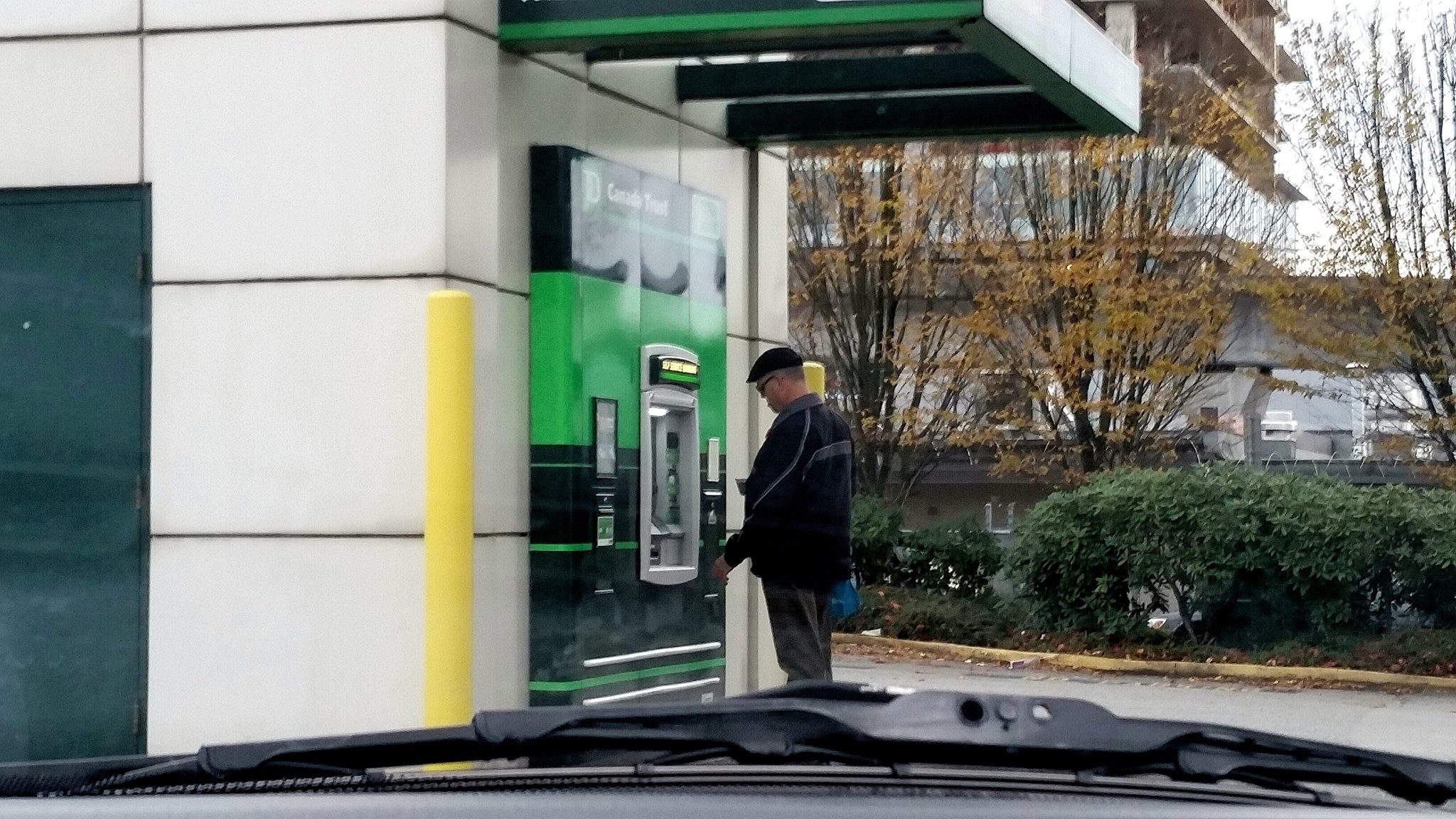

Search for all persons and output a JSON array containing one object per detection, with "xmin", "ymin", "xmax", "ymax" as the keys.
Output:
[{"xmin": 713, "ymin": 346, "xmax": 858, "ymax": 682}]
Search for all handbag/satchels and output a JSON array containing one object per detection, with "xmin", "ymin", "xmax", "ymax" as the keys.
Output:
[{"xmin": 827, "ymin": 580, "xmax": 862, "ymax": 625}]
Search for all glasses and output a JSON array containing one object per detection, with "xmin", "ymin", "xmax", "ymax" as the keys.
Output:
[{"xmin": 755, "ymin": 376, "xmax": 774, "ymax": 397}]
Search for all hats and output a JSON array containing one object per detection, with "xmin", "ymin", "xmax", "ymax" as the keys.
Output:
[{"xmin": 746, "ymin": 347, "xmax": 803, "ymax": 383}]
